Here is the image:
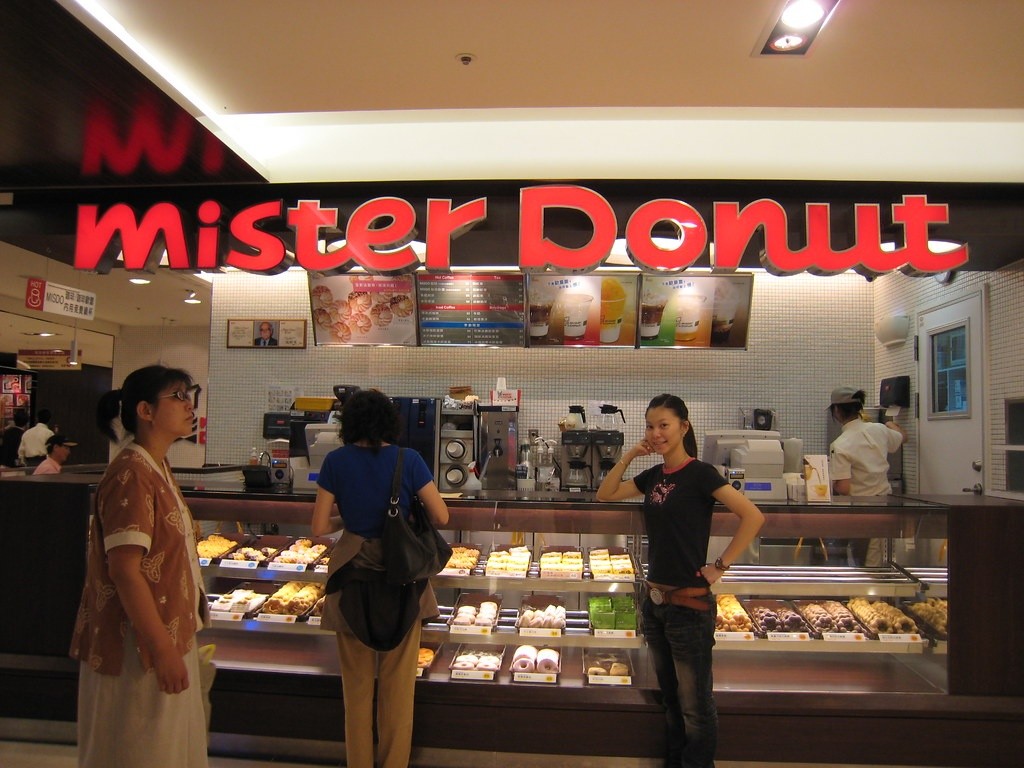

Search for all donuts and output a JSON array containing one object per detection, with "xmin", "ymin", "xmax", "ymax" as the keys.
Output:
[
  {"xmin": 518, "ymin": 604, "xmax": 566, "ymax": 631},
  {"xmin": 262, "ymin": 581, "xmax": 326, "ymax": 617},
  {"xmin": 417, "ymin": 647, "xmax": 434, "ymax": 667},
  {"xmin": 311, "ymin": 275, "xmax": 415, "ymax": 342},
  {"xmin": 197, "ymin": 534, "xmax": 331, "ymax": 566},
  {"xmin": 714, "ymin": 594, "xmax": 917, "ymax": 637},
  {"xmin": 512, "ymin": 644, "xmax": 560, "ymax": 674},
  {"xmin": 453, "ymin": 654, "xmax": 500, "ymax": 671},
  {"xmin": 912, "ymin": 597, "xmax": 949, "ymax": 636},
  {"xmin": 454, "ymin": 601, "xmax": 498, "ymax": 627}
]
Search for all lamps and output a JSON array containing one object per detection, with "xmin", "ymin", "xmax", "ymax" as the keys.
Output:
[
  {"xmin": 188, "ymin": 292, "xmax": 196, "ymax": 297},
  {"xmin": 69, "ymin": 270, "xmax": 82, "ymax": 364},
  {"xmin": 873, "ymin": 314, "xmax": 910, "ymax": 348}
]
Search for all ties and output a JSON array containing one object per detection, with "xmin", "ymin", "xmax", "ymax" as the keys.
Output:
[{"xmin": 262, "ymin": 340, "xmax": 267, "ymax": 347}]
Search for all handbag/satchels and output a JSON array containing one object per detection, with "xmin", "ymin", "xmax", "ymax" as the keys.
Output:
[{"xmin": 386, "ymin": 446, "xmax": 453, "ymax": 585}]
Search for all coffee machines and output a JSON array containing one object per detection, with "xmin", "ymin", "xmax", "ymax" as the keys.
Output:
[
  {"xmin": 593, "ymin": 431, "xmax": 624, "ymax": 491},
  {"xmin": 561, "ymin": 431, "xmax": 592, "ymax": 491}
]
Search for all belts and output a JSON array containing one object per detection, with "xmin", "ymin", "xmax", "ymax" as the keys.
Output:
[{"xmin": 642, "ymin": 580, "xmax": 711, "ymax": 610}]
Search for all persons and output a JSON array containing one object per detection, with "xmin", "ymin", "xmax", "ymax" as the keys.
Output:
[
  {"xmin": 253, "ymin": 321, "xmax": 277, "ymax": 346},
  {"xmin": 311, "ymin": 391, "xmax": 449, "ymax": 768},
  {"xmin": 595, "ymin": 395, "xmax": 767, "ymax": 768},
  {"xmin": 821, "ymin": 385, "xmax": 909, "ymax": 566},
  {"xmin": 68, "ymin": 363, "xmax": 211, "ymax": 768},
  {"xmin": 0, "ymin": 407, "xmax": 59, "ymax": 468},
  {"xmin": 31, "ymin": 434, "xmax": 78, "ymax": 475}
]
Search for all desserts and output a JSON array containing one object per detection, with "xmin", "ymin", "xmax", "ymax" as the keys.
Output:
[{"xmin": 588, "ymin": 663, "xmax": 629, "ymax": 676}]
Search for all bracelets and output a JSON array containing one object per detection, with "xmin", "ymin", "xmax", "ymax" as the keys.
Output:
[{"xmin": 619, "ymin": 459, "xmax": 629, "ymax": 466}]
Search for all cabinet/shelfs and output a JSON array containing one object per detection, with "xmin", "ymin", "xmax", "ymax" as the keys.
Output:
[{"xmin": 80, "ymin": 480, "xmax": 1024, "ymax": 768}]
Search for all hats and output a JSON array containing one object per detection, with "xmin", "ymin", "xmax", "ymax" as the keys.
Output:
[
  {"xmin": 824, "ymin": 384, "xmax": 862, "ymax": 410},
  {"xmin": 44, "ymin": 435, "xmax": 78, "ymax": 448}
]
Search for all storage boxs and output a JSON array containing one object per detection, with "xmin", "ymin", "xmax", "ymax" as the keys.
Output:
[
  {"xmin": 509, "ymin": 645, "xmax": 563, "ymax": 678},
  {"xmin": 791, "ymin": 599, "xmax": 875, "ymax": 639},
  {"xmin": 538, "ymin": 545, "xmax": 585, "ymax": 579},
  {"xmin": 802, "ymin": 462, "xmax": 833, "ymax": 506},
  {"xmin": 515, "ymin": 594, "xmax": 566, "ymax": 634},
  {"xmin": 417, "ymin": 641, "xmax": 445, "ymax": 674},
  {"xmin": 483, "ymin": 543, "xmax": 534, "ymax": 574},
  {"xmin": 445, "ymin": 592, "xmax": 503, "ymax": 633},
  {"xmin": 449, "ymin": 643, "xmax": 507, "ymax": 678},
  {"xmin": 711, "ymin": 594, "xmax": 759, "ymax": 636},
  {"xmin": 449, "ymin": 386, "xmax": 471, "ymax": 400},
  {"xmin": 294, "ymin": 397, "xmax": 338, "ymax": 410},
  {"xmin": 212, "ymin": 581, "xmax": 279, "ymax": 618},
  {"xmin": 489, "ymin": 389, "xmax": 521, "ymax": 407},
  {"xmin": 266, "ymin": 536, "xmax": 337, "ymax": 570},
  {"xmin": 444, "ymin": 543, "xmax": 483, "ymax": 576},
  {"xmin": 954, "ymin": 379, "xmax": 966, "ymax": 409},
  {"xmin": 253, "ymin": 581, "xmax": 317, "ymax": 619},
  {"xmin": 219, "ymin": 535, "xmax": 296, "ymax": 567},
  {"xmin": 588, "ymin": 613, "xmax": 639, "ymax": 636},
  {"xmin": 312, "ymin": 542, "xmax": 338, "ymax": 570},
  {"xmin": 742, "ymin": 598, "xmax": 818, "ymax": 639},
  {"xmin": 842, "ymin": 600, "xmax": 926, "ymax": 638},
  {"xmin": 587, "ymin": 545, "xmax": 639, "ymax": 583},
  {"xmin": 582, "ymin": 647, "xmax": 635, "ymax": 679},
  {"xmin": 197, "ymin": 533, "xmax": 255, "ymax": 565}
]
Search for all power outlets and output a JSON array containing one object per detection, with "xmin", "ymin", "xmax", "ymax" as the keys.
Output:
[{"xmin": 528, "ymin": 428, "xmax": 539, "ymax": 446}]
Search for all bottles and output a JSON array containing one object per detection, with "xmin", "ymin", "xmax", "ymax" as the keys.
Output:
[
  {"xmin": 249, "ymin": 447, "xmax": 259, "ymax": 465},
  {"xmin": 937, "ymin": 345, "xmax": 947, "ymax": 369},
  {"xmin": 517, "ymin": 435, "xmax": 537, "ymax": 491}
]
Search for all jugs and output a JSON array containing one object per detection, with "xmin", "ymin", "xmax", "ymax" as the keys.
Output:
[
  {"xmin": 596, "ymin": 458, "xmax": 623, "ymax": 488},
  {"xmin": 565, "ymin": 405, "xmax": 588, "ymax": 430},
  {"xmin": 596, "ymin": 404, "xmax": 626, "ymax": 431},
  {"xmin": 566, "ymin": 460, "xmax": 593, "ymax": 489}
]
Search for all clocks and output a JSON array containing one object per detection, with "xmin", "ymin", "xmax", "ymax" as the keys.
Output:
[{"xmin": 934, "ymin": 271, "xmax": 954, "ymax": 284}]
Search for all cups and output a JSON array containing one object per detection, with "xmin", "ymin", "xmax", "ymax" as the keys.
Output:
[
  {"xmin": 600, "ymin": 298, "xmax": 628, "ymax": 343},
  {"xmin": 678, "ymin": 294, "xmax": 708, "ymax": 339},
  {"xmin": 713, "ymin": 297, "xmax": 739, "ymax": 337},
  {"xmin": 565, "ymin": 294, "xmax": 593, "ymax": 341},
  {"xmin": 496, "ymin": 377, "xmax": 507, "ymax": 391},
  {"xmin": 530, "ymin": 290, "xmax": 555, "ymax": 341},
  {"xmin": 642, "ymin": 298, "xmax": 668, "ymax": 341}
]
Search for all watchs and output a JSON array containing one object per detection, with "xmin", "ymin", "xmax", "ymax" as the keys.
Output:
[{"xmin": 714, "ymin": 557, "xmax": 730, "ymax": 571}]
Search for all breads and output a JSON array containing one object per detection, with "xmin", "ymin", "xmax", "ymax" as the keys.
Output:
[{"xmin": 443, "ymin": 547, "xmax": 636, "ymax": 575}]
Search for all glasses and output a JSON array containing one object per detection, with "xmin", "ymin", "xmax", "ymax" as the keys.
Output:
[
  {"xmin": 157, "ymin": 390, "xmax": 192, "ymax": 402},
  {"xmin": 259, "ymin": 327, "xmax": 271, "ymax": 332}
]
